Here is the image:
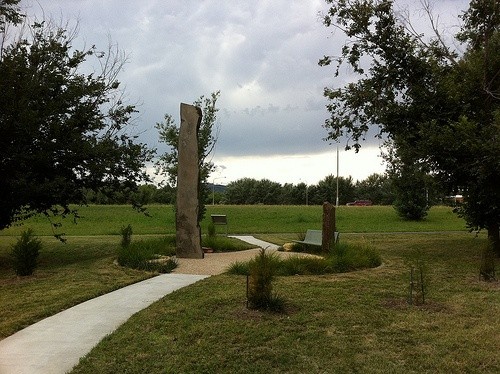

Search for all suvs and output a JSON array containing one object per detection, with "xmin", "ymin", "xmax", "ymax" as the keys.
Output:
[{"xmin": 347, "ymin": 200, "xmax": 372, "ymax": 206}]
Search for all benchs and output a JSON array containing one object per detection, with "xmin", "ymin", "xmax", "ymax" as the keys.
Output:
[{"xmin": 292, "ymin": 230, "xmax": 340, "ymax": 253}]
